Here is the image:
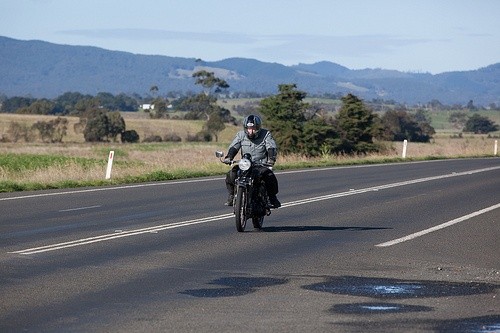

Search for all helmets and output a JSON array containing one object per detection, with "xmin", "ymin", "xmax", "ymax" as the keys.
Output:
[{"xmin": 243, "ymin": 114, "xmax": 262, "ymax": 139}]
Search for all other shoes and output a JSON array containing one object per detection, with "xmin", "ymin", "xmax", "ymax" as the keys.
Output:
[
  {"xmin": 269, "ymin": 194, "xmax": 281, "ymax": 207},
  {"xmin": 224, "ymin": 194, "xmax": 234, "ymax": 206}
]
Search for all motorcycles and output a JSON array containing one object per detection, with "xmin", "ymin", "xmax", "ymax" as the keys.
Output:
[{"xmin": 214, "ymin": 150, "xmax": 278, "ymax": 232}]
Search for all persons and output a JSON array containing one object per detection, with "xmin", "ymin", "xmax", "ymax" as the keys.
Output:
[{"xmin": 224, "ymin": 114, "xmax": 281, "ymax": 207}]
